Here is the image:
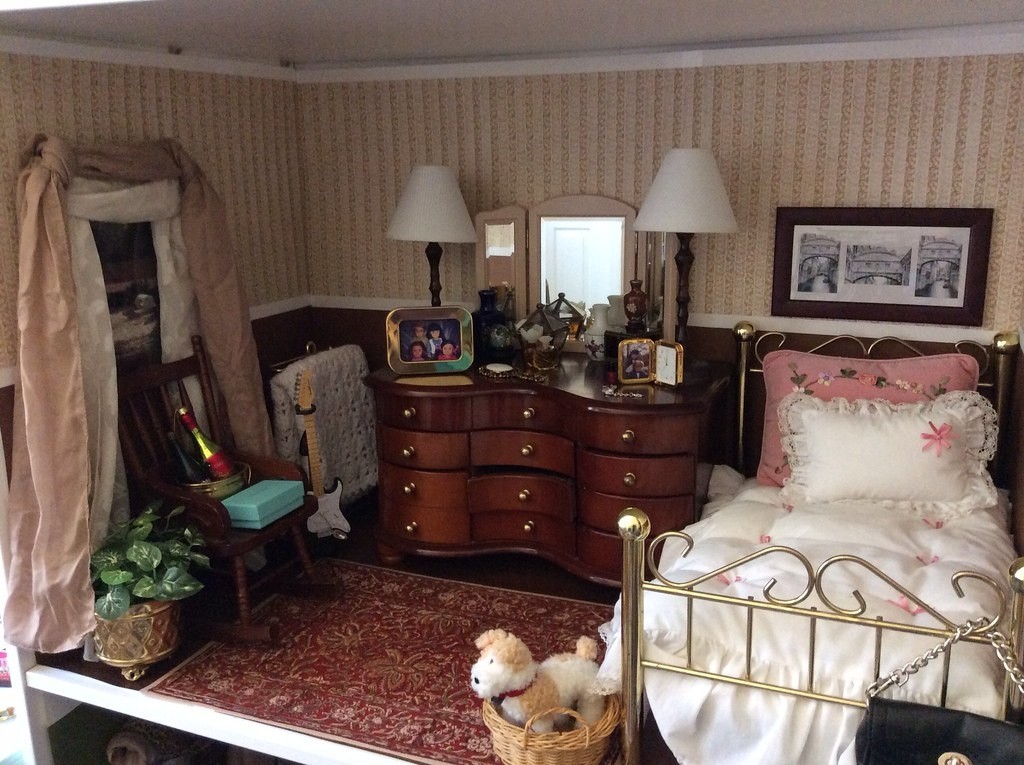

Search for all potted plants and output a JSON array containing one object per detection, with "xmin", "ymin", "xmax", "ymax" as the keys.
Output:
[{"xmin": 83, "ymin": 498, "xmax": 212, "ymax": 682}]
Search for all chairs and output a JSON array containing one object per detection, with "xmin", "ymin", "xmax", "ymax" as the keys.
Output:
[{"xmin": 115, "ymin": 333, "xmax": 343, "ymax": 644}]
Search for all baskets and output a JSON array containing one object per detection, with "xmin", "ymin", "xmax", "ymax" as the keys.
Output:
[{"xmin": 482, "ymin": 675, "xmax": 622, "ymax": 765}]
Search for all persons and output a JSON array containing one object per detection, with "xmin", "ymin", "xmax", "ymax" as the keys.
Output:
[{"xmin": 623, "ymin": 350, "xmax": 649, "ymax": 379}]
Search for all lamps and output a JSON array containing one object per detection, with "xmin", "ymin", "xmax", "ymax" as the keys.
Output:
[
  {"xmin": 387, "ymin": 165, "xmax": 481, "ymax": 307},
  {"xmin": 630, "ymin": 149, "xmax": 741, "ymax": 385}
]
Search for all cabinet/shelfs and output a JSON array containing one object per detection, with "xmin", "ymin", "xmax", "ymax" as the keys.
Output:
[{"xmin": 370, "ymin": 355, "xmax": 730, "ymax": 591}]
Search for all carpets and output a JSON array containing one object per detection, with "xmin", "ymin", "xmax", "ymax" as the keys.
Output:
[{"xmin": 134, "ymin": 556, "xmax": 617, "ymax": 765}]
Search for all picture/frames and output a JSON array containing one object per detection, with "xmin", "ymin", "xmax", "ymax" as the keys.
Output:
[
  {"xmin": 615, "ymin": 338, "xmax": 683, "ymax": 388},
  {"xmin": 385, "ymin": 304, "xmax": 475, "ymax": 375},
  {"xmin": 87, "ymin": 220, "xmax": 162, "ymax": 356},
  {"xmin": 770, "ymin": 207, "xmax": 995, "ymax": 327}
]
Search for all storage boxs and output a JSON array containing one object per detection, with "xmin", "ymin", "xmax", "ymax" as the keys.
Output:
[{"xmin": 219, "ymin": 479, "xmax": 304, "ymax": 529}]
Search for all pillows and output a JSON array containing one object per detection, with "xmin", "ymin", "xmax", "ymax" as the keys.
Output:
[
  {"xmin": 776, "ymin": 389, "xmax": 998, "ymax": 520},
  {"xmin": 754, "ymin": 351, "xmax": 981, "ymax": 488}
]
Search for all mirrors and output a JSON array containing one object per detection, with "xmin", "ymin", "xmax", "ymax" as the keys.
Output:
[
  {"xmin": 527, "ymin": 195, "xmax": 638, "ymax": 355},
  {"xmin": 646, "ymin": 231, "xmax": 679, "ymax": 340},
  {"xmin": 476, "ymin": 205, "xmax": 527, "ymax": 320}
]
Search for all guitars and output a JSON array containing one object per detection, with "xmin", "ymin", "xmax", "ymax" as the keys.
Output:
[{"xmin": 294, "ymin": 369, "xmax": 352, "ymax": 541}]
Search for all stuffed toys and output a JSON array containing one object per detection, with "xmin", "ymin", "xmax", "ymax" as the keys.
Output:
[{"xmin": 470, "ymin": 628, "xmax": 607, "ymax": 734}]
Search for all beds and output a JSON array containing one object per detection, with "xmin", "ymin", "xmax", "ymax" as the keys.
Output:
[{"xmin": 587, "ymin": 322, "xmax": 1024, "ymax": 765}]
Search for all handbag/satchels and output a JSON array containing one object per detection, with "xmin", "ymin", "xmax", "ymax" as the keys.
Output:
[{"xmin": 855, "ymin": 618, "xmax": 1024, "ymax": 765}]
